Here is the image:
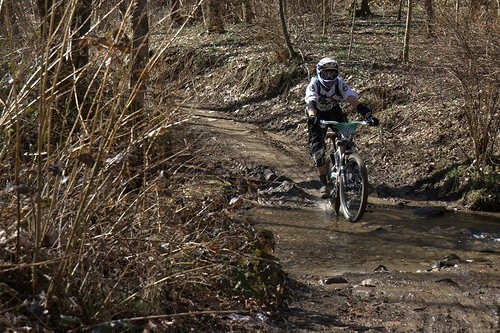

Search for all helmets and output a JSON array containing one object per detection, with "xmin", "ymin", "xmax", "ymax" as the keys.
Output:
[{"xmin": 317, "ymin": 57, "xmax": 338, "ymax": 89}]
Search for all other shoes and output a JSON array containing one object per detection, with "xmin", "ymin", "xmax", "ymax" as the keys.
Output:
[{"xmin": 320, "ymin": 185, "xmax": 330, "ymax": 199}]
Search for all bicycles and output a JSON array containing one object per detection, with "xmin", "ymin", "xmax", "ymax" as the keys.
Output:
[{"xmin": 309, "ymin": 116, "xmax": 379, "ymax": 222}]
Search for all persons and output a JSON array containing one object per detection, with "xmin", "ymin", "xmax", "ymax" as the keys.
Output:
[{"xmin": 303, "ymin": 57, "xmax": 380, "ymax": 199}]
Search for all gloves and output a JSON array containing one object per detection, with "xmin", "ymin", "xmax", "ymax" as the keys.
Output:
[
  {"xmin": 367, "ymin": 114, "xmax": 379, "ymax": 127},
  {"xmin": 308, "ymin": 115, "xmax": 317, "ymax": 128}
]
